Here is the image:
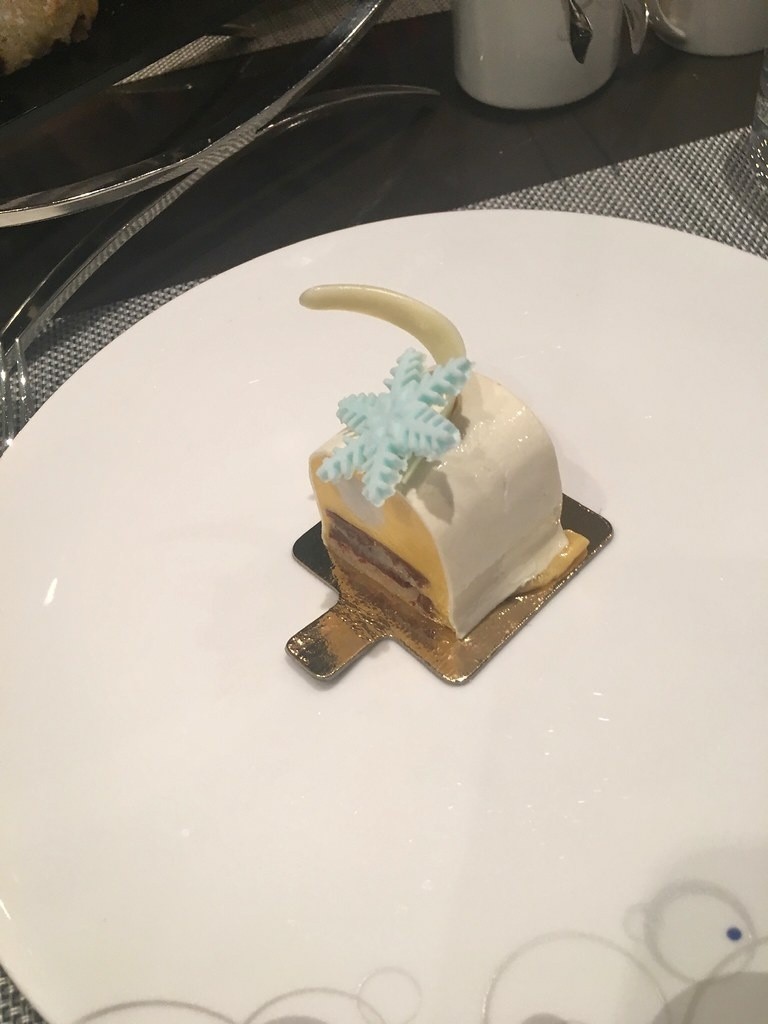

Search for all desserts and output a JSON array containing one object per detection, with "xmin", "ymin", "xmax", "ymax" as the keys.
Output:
[{"xmin": 295, "ymin": 284, "xmax": 588, "ymax": 638}]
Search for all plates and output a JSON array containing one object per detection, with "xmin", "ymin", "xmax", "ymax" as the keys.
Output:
[{"xmin": 3, "ymin": 210, "xmax": 766, "ymax": 1020}]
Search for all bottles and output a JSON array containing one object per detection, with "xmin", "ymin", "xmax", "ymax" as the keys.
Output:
[{"xmin": 744, "ymin": 50, "xmax": 768, "ymax": 194}]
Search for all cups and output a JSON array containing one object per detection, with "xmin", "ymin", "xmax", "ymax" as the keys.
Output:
[
  {"xmin": 649, "ymin": 0, "xmax": 767, "ymax": 56},
  {"xmin": 450, "ymin": 0, "xmax": 648, "ymax": 112}
]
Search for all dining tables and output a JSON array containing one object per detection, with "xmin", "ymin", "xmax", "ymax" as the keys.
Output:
[{"xmin": 0, "ymin": 3, "xmax": 765, "ymax": 1024}]
